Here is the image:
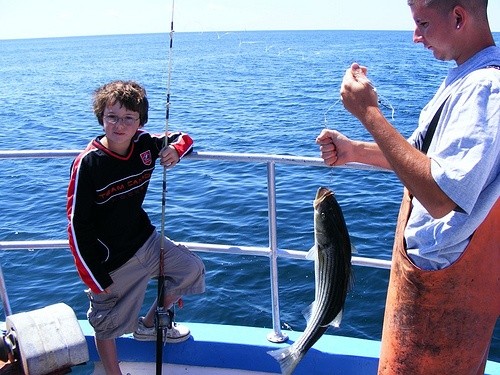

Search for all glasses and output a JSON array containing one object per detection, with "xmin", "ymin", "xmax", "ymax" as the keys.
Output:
[{"xmin": 103, "ymin": 112, "xmax": 140, "ymax": 125}]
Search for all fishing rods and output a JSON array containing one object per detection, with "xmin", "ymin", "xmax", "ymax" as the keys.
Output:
[{"xmin": 150, "ymin": 0, "xmax": 178, "ymax": 375}]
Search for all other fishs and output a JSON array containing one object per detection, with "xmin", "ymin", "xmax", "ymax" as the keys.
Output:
[{"xmin": 266, "ymin": 187, "xmax": 358, "ymax": 375}]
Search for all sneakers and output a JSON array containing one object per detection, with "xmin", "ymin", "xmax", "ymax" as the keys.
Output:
[{"xmin": 133, "ymin": 317, "xmax": 191, "ymax": 344}]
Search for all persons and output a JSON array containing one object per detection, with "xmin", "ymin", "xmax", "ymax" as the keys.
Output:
[
  {"xmin": 66, "ymin": 80, "xmax": 206, "ymax": 375},
  {"xmin": 315, "ymin": 0, "xmax": 500, "ymax": 375}
]
